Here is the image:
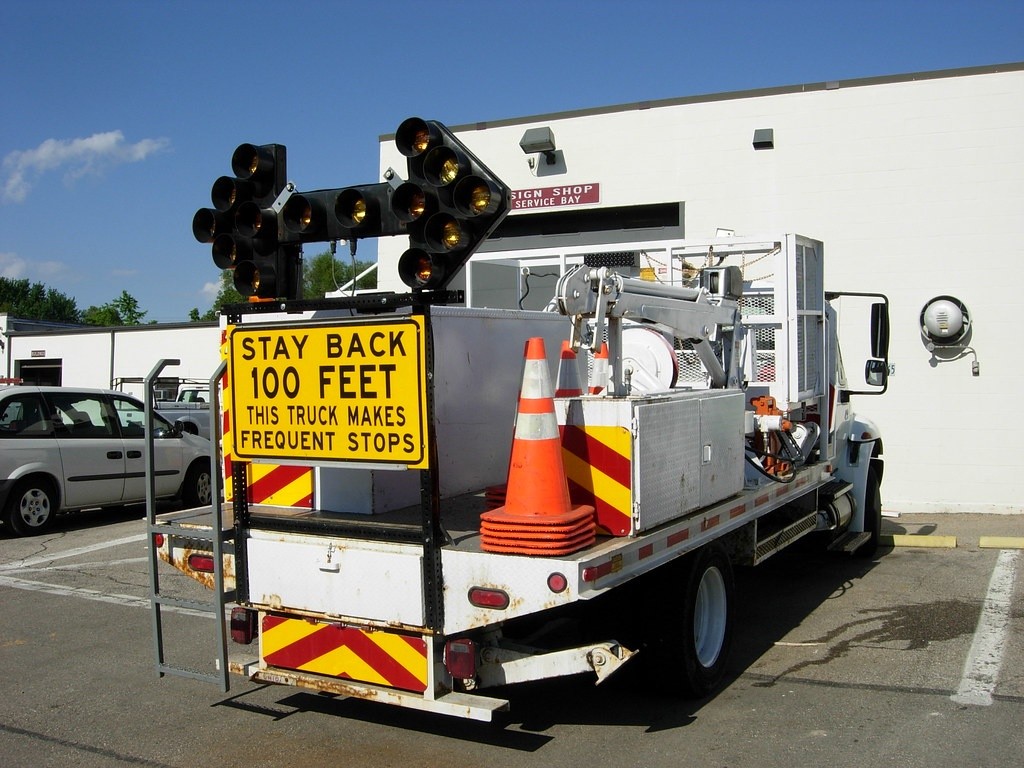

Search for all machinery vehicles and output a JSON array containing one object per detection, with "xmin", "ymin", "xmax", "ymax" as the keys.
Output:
[{"xmin": 144, "ymin": 117, "xmax": 889, "ymax": 725}]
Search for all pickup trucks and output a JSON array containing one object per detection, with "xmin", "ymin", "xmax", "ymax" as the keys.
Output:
[{"xmin": 111, "ymin": 377, "xmax": 223, "ymax": 442}]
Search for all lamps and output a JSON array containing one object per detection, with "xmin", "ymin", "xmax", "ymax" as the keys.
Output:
[{"xmin": 519, "ymin": 126, "xmax": 556, "ymax": 165}]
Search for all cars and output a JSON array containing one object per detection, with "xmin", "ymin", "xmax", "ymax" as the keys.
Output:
[{"xmin": 0, "ymin": 384, "xmax": 221, "ymax": 535}]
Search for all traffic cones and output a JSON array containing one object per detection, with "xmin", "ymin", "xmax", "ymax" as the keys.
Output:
[
  {"xmin": 481, "ymin": 335, "xmax": 596, "ymax": 557},
  {"xmin": 588, "ymin": 341, "xmax": 609, "ymax": 395},
  {"xmin": 554, "ymin": 340, "xmax": 583, "ymax": 397}
]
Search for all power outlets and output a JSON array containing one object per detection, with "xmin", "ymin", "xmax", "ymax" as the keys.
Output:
[{"xmin": 528, "ymin": 157, "xmax": 534, "ymax": 167}]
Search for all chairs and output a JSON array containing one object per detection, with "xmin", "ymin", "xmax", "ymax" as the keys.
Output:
[
  {"xmin": 194, "ymin": 397, "xmax": 205, "ymax": 403},
  {"xmin": 9, "ymin": 420, "xmax": 50, "ymax": 430},
  {"xmin": 52, "ymin": 406, "xmax": 103, "ymax": 437}
]
